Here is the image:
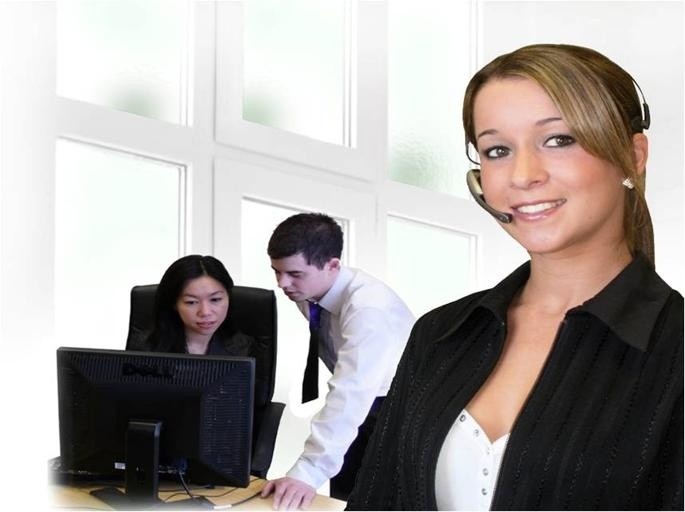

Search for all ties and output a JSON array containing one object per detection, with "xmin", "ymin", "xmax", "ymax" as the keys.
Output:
[{"xmin": 300, "ymin": 302, "xmax": 320, "ymax": 404}]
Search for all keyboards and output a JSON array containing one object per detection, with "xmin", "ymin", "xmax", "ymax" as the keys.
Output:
[{"xmin": 49, "ymin": 455, "xmax": 181, "ymax": 487}]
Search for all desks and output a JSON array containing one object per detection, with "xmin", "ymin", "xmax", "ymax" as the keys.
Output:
[{"xmin": 45, "ymin": 454, "xmax": 352, "ymax": 511}]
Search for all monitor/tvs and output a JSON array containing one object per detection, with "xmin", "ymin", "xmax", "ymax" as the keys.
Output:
[{"xmin": 56, "ymin": 346, "xmax": 256, "ymax": 510}]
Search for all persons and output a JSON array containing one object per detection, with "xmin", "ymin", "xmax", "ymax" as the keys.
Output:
[
  {"xmin": 133, "ymin": 253, "xmax": 270, "ymax": 471},
  {"xmin": 344, "ymin": 43, "xmax": 685, "ymax": 511},
  {"xmin": 260, "ymin": 211, "xmax": 417, "ymax": 511}
]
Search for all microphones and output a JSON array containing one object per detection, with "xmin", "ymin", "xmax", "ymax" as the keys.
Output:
[{"xmin": 467, "ymin": 169, "xmax": 513, "ymax": 225}]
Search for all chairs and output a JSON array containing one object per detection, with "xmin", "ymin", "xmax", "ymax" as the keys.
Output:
[{"xmin": 124, "ymin": 283, "xmax": 289, "ymax": 480}]
